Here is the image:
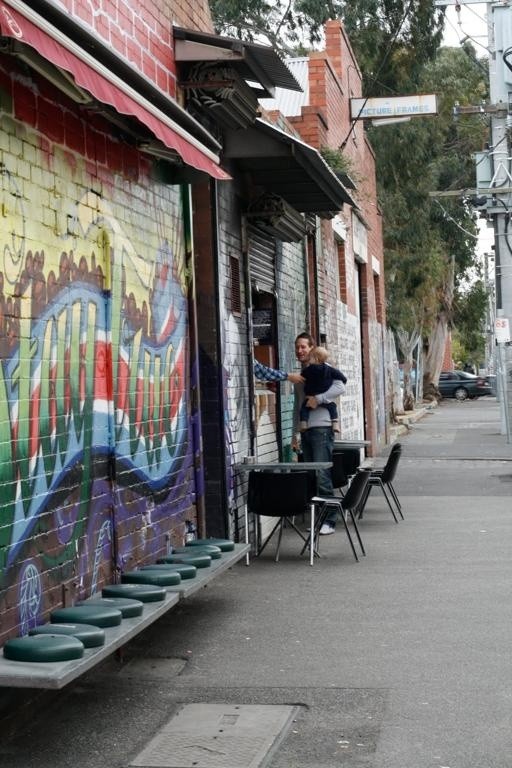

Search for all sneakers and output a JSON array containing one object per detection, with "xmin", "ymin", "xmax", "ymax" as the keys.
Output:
[
  {"xmin": 332, "ymin": 421, "xmax": 340, "ymax": 433},
  {"xmin": 306, "ymin": 522, "xmax": 335, "ymax": 535},
  {"xmin": 299, "ymin": 421, "xmax": 306, "ymax": 433}
]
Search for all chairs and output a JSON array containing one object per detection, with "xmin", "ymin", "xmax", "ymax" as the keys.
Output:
[{"xmin": 244, "ymin": 437, "xmax": 404, "ymax": 567}]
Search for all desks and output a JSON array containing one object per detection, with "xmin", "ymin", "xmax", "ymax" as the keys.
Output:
[{"xmin": 232, "ymin": 462, "xmax": 335, "ymax": 562}]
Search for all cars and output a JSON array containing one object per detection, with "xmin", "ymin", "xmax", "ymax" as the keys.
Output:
[{"xmin": 412, "ymin": 370, "xmax": 494, "ymax": 401}]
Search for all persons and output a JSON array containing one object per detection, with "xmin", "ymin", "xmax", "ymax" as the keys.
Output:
[
  {"xmin": 253, "ymin": 358, "xmax": 308, "ymax": 385},
  {"xmin": 289, "ymin": 332, "xmax": 347, "ymax": 535},
  {"xmin": 298, "ymin": 346, "xmax": 347, "ymax": 433}
]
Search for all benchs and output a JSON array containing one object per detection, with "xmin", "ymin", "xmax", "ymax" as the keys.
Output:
[{"xmin": 0, "ymin": 537, "xmax": 252, "ymax": 687}]
